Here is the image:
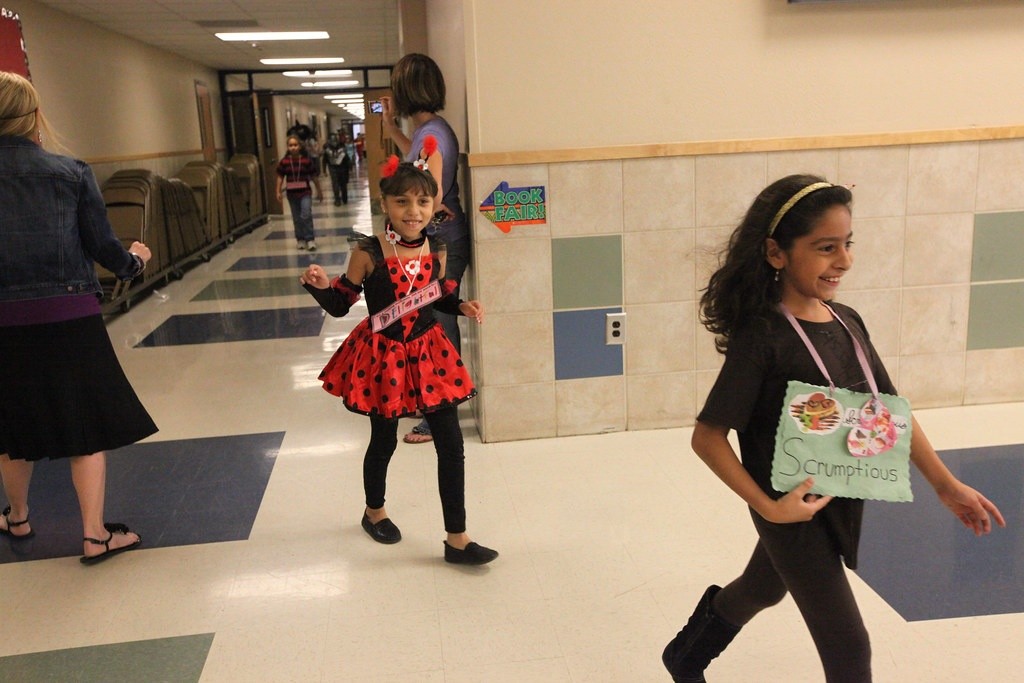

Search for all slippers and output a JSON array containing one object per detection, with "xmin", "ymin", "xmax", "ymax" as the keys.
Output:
[{"xmin": 403, "ymin": 420, "xmax": 434, "ymax": 444}]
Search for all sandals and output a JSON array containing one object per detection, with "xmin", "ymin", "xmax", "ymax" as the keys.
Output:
[
  {"xmin": 80, "ymin": 522, "xmax": 142, "ymax": 565},
  {"xmin": 0, "ymin": 506, "xmax": 36, "ymax": 540}
]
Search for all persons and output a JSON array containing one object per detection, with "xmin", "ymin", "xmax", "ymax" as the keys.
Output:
[
  {"xmin": 299, "ymin": 153, "xmax": 498, "ymax": 565},
  {"xmin": 322, "ymin": 127, "xmax": 367, "ymax": 206},
  {"xmin": 275, "ymin": 134, "xmax": 323, "ymax": 251},
  {"xmin": 662, "ymin": 174, "xmax": 1006, "ymax": 683},
  {"xmin": 0, "ymin": 69, "xmax": 159, "ymax": 564},
  {"xmin": 379, "ymin": 53, "xmax": 471, "ymax": 444}
]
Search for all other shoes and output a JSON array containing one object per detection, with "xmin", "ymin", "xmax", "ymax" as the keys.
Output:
[{"xmin": 336, "ymin": 202, "xmax": 340, "ymax": 206}]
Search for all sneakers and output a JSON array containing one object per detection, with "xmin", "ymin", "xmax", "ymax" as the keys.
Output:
[
  {"xmin": 297, "ymin": 239, "xmax": 306, "ymax": 249},
  {"xmin": 306, "ymin": 240, "xmax": 316, "ymax": 250}
]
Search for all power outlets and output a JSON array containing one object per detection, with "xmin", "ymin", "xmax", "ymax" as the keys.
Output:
[{"xmin": 605, "ymin": 313, "xmax": 626, "ymax": 346}]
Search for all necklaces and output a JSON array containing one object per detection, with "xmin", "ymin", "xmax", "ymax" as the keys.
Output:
[
  {"xmin": 385, "ymin": 223, "xmax": 427, "ymax": 248},
  {"xmin": 388, "ymin": 240, "xmax": 425, "ymax": 297}
]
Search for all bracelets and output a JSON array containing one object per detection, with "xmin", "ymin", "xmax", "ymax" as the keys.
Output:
[{"xmin": 134, "ymin": 253, "xmax": 145, "ymax": 276}]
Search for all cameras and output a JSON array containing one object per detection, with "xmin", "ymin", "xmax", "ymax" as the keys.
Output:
[{"xmin": 367, "ymin": 100, "xmax": 383, "ymax": 115}]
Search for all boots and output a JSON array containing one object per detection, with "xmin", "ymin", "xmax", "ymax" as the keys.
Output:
[{"xmin": 662, "ymin": 583, "xmax": 743, "ymax": 683}]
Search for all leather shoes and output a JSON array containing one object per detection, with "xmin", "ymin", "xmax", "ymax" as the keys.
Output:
[
  {"xmin": 443, "ymin": 539, "xmax": 498, "ymax": 565},
  {"xmin": 361, "ymin": 507, "xmax": 401, "ymax": 544}
]
